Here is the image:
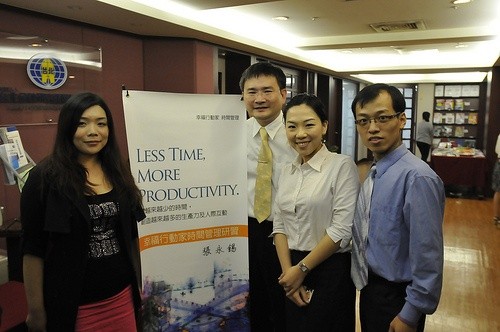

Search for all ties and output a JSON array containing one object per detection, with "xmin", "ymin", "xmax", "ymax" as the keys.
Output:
[
  {"xmin": 350, "ymin": 166, "xmax": 377, "ymax": 291},
  {"xmin": 252, "ymin": 127, "xmax": 273, "ymax": 223}
]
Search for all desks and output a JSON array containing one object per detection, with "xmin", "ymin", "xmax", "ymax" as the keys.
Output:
[{"xmin": 432, "ymin": 147, "xmax": 486, "ymax": 198}]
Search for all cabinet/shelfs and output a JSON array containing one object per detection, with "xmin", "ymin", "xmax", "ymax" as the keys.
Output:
[{"xmin": 433, "ymin": 84, "xmax": 480, "ymax": 140}]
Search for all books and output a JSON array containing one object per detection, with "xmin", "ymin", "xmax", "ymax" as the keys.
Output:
[{"xmin": 433, "ymin": 98, "xmax": 479, "ymax": 156}]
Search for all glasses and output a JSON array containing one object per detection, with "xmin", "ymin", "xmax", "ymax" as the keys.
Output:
[{"xmin": 354, "ymin": 112, "xmax": 401, "ymax": 127}]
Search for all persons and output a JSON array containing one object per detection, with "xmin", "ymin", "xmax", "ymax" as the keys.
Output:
[
  {"xmin": 240, "ymin": 62, "xmax": 299, "ymax": 332},
  {"xmin": 350, "ymin": 83, "xmax": 446, "ymax": 332},
  {"xmin": 268, "ymin": 92, "xmax": 361, "ymax": 332},
  {"xmin": 493, "ymin": 134, "xmax": 500, "ymax": 227},
  {"xmin": 20, "ymin": 92, "xmax": 147, "ymax": 332},
  {"xmin": 416, "ymin": 111, "xmax": 433, "ymax": 162}
]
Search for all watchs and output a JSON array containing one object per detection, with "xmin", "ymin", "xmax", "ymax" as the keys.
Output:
[{"xmin": 298, "ymin": 261, "xmax": 311, "ymax": 273}]
